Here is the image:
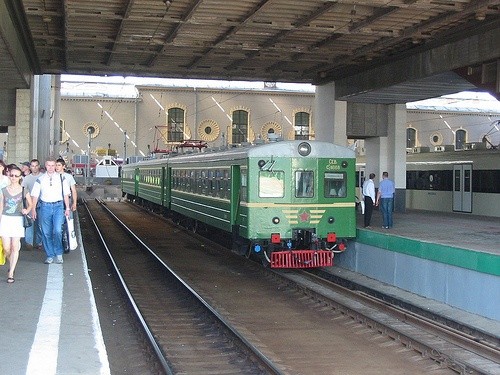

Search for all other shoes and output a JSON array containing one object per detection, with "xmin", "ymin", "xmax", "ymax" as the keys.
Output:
[
  {"xmin": 365, "ymin": 226, "xmax": 373, "ymax": 229},
  {"xmin": 43, "ymin": 257, "xmax": 54, "ymax": 264},
  {"xmin": 56, "ymin": 254, "xmax": 64, "ymax": 264},
  {"xmin": 7, "ymin": 277, "xmax": 15, "ymax": 283}
]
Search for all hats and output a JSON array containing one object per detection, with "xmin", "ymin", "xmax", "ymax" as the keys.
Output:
[{"xmin": 20, "ymin": 162, "xmax": 31, "ymax": 168}]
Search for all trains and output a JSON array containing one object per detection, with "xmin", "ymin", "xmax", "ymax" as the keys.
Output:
[
  {"xmin": 355, "ymin": 118, "xmax": 500, "ymax": 221},
  {"xmin": 116, "ymin": 134, "xmax": 360, "ymax": 271}
]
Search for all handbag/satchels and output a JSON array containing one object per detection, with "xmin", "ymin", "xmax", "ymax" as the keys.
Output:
[
  {"xmin": 61, "ymin": 216, "xmax": 78, "ymax": 252},
  {"xmin": 22, "ymin": 186, "xmax": 33, "ymax": 228},
  {"xmin": 0, "ymin": 238, "xmax": 6, "ymax": 266},
  {"xmin": 361, "ymin": 201, "xmax": 365, "ymax": 215}
]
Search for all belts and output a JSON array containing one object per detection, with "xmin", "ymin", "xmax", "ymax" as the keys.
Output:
[{"xmin": 40, "ymin": 201, "xmax": 61, "ymax": 204}]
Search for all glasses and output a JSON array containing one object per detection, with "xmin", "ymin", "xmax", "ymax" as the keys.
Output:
[{"xmin": 9, "ymin": 174, "xmax": 20, "ymax": 177}]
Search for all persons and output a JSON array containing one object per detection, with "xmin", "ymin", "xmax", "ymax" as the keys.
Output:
[
  {"xmin": 0, "ymin": 157, "xmax": 80, "ymax": 283},
  {"xmin": 376, "ymin": 172, "xmax": 396, "ymax": 229},
  {"xmin": 363, "ymin": 173, "xmax": 376, "ymax": 230}
]
